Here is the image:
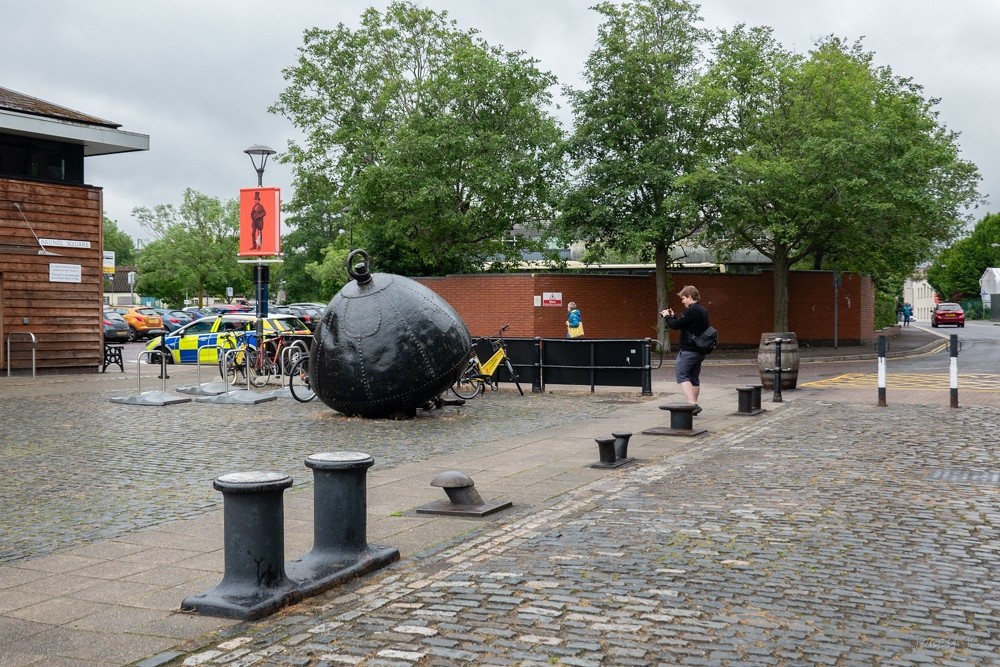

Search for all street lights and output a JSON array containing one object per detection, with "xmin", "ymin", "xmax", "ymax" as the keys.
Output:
[
  {"xmin": 243, "ymin": 143, "xmax": 277, "ymax": 377},
  {"xmin": 342, "ymin": 205, "xmax": 360, "ymax": 271}
]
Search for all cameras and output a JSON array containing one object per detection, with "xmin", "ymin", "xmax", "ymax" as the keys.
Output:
[{"xmin": 660, "ymin": 310, "xmax": 672, "ymax": 318}]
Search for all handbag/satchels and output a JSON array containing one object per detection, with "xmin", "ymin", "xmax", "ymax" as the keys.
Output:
[
  {"xmin": 689, "ymin": 326, "xmax": 718, "ymax": 353},
  {"xmin": 566, "ymin": 321, "xmax": 584, "ymax": 338}
]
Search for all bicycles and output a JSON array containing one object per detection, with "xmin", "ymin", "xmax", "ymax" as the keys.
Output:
[
  {"xmin": 449, "ymin": 324, "xmax": 526, "ymax": 399},
  {"xmin": 288, "ymin": 355, "xmax": 318, "ymax": 403},
  {"xmin": 245, "ymin": 328, "xmax": 303, "ymax": 388},
  {"xmin": 218, "ymin": 321, "xmax": 259, "ymax": 386}
]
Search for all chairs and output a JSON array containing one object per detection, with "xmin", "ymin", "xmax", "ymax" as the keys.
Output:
[{"xmin": 102, "ymin": 332, "xmax": 124, "ymax": 372}]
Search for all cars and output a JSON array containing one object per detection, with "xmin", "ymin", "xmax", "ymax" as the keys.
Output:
[
  {"xmin": 110, "ymin": 305, "xmax": 166, "ymax": 343},
  {"xmin": 101, "ymin": 310, "xmax": 131, "ymax": 344},
  {"xmin": 155, "ymin": 305, "xmax": 257, "ymax": 335},
  {"xmin": 929, "ymin": 303, "xmax": 966, "ymax": 327},
  {"xmin": 252, "ymin": 300, "xmax": 327, "ymax": 332},
  {"xmin": 145, "ymin": 305, "xmax": 315, "ymax": 365}
]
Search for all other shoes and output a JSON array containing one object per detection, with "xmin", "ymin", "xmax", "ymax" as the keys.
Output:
[{"xmin": 694, "ymin": 403, "xmax": 702, "ymax": 416}]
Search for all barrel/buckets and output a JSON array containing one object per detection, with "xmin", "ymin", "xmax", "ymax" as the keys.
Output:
[{"xmin": 757, "ymin": 331, "xmax": 799, "ymax": 390}]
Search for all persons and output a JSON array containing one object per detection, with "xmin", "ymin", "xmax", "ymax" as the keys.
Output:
[
  {"xmin": 250, "ymin": 192, "xmax": 266, "ymax": 250},
  {"xmin": 659, "ymin": 286, "xmax": 711, "ymax": 416},
  {"xmin": 566, "ymin": 301, "xmax": 585, "ymax": 339},
  {"xmin": 895, "ymin": 297, "xmax": 914, "ymax": 327}
]
